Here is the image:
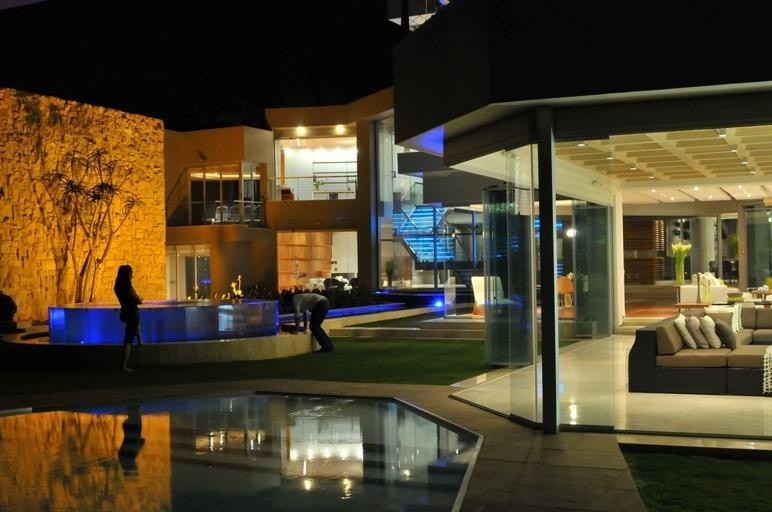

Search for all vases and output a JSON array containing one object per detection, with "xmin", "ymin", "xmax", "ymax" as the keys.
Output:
[{"xmin": 675, "ymin": 251, "xmax": 685, "ymax": 286}]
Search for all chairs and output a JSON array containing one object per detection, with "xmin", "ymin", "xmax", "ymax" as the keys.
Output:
[{"xmin": 201, "ymin": 202, "xmax": 264, "ymax": 227}]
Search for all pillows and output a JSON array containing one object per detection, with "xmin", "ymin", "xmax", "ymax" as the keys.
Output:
[
  {"xmin": 691, "ymin": 270, "xmax": 725, "ymax": 285},
  {"xmin": 674, "ymin": 312, "xmax": 738, "ymax": 350}
]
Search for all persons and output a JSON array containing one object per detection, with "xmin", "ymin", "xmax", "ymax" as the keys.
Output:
[
  {"xmin": 283, "ymin": 291, "xmax": 333, "ymax": 352},
  {"xmin": 118, "ymin": 403, "xmax": 146, "ymax": 484},
  {"xmin": 114, "ymin": 265, "xmax": 143, "ymax": 372}
]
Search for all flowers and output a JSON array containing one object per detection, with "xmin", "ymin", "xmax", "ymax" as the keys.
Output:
[{"xmin": 671, "ymin": 241, "xmax": 693, "ymax": 270}]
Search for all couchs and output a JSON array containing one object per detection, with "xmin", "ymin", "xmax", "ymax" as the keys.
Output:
[
  {"xmin": 680, "ymin": 283, "xmax": 740, "ymax": 311},
  {"xmin": 470, "ymin": 275, "xmax": 519, "ymax": 317},
  {"xmin": 628, "ymin": 308, "xmax": 772, "ymax": 398}
]
